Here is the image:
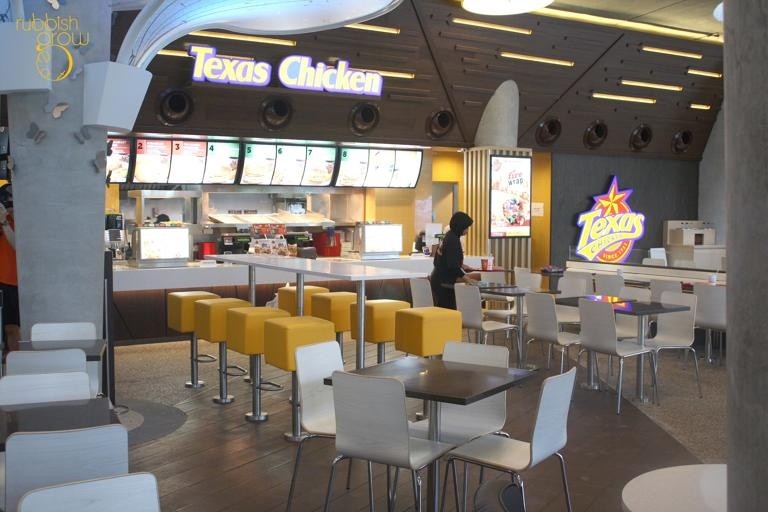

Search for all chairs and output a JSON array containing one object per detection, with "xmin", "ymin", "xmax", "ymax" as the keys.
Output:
[
  {"xmin": 5, "ymin": 422, "xmax": 129, "ymax": 512},
  {"xmin": 5, "ymin": 349, "xmax": 87, "ymax": 373},
  {"xmin": 31, "ymin": 322, "xmax": 99, "ymax": 399},
  {"xmin": 17, "ymin": 471, "xmax": 161, "ymax": 512},
  {"xmin": 0, "ymin": 371, "xmax": 90, "ymax": 401}
]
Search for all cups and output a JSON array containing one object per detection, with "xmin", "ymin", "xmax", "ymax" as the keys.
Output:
[
  {"xmin": 617, "ymin": 269, "xmax": 624, "ymax": 278},
  {"xmin": 708, "ymin": 275, "xmax": 717, "ymax": 287},
  {"xmin": 481, "ymin": 256, "xmax": 494, "ymax": 271}
]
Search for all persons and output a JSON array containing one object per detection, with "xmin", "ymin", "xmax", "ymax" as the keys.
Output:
[
  {"xmin": 430, "ymin": 212, "xmax": 476, "ymax": 310},
  {"xmin": 0, "ymin": 184, "xmax": 20, "ymax": 351},
  {"xmin": 415, "ymin": 231, "xmax": 426, "ymax": 252}
]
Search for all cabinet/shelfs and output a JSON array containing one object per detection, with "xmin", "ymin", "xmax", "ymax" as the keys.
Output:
[{"xmin": 662, "ymin": 219, "xmax": 727, "ymax": 271}]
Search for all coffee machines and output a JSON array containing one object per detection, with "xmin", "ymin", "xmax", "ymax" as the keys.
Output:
[{"xmin": 104, "ymin": 214, "xmax": 127, "ymax": 260}]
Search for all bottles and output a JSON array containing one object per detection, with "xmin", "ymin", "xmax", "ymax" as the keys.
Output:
[{"xmin": 254, "ymin": 240, "xmax": 260, "ymax": 256}]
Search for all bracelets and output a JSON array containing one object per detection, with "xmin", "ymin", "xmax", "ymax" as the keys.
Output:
[{"xmin": 0, "ymin": 219, "xmax": 9, "ymax": 225}]
